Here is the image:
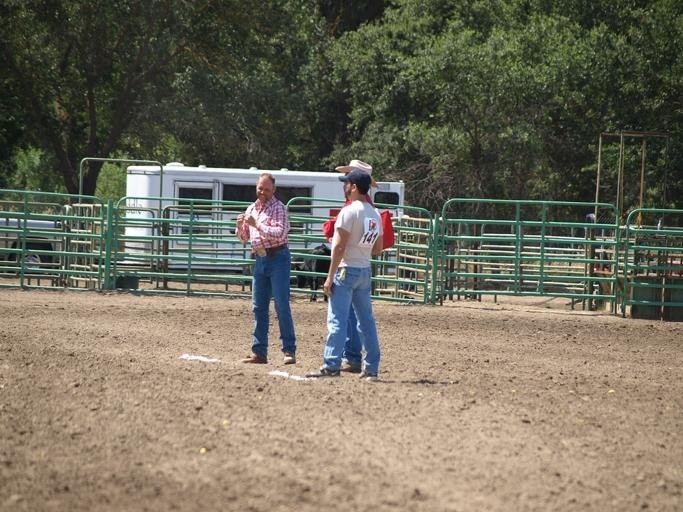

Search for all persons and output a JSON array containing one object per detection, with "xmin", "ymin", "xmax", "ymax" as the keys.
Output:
[
  {"xmin": 306, "ymin": 168, "xmax": 386, "ymax": 380},
  {"xmin": 233, "ymin": 172, "xmax": 297, "ymax": 365},
  {"xmin": 322, "ymin": 159, "xmax": 377, "ymax": 373}
]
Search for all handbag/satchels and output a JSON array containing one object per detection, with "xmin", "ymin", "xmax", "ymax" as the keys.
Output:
[{"xmin": 323, "ymin": 210, "xmax": 395, "ymax": 254}]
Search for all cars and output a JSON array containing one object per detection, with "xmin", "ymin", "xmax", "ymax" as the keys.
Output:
[{"xmin": 0, "ymin": 218, "xmax": 60, "ymax": 274}]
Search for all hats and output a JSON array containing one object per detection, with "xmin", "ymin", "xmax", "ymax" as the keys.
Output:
[{"xmin": 335, "ymin": 160, "xmax": 379, "ymax": 192}]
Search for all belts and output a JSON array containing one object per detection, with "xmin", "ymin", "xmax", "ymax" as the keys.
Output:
[{"xmin": 252, "ymin": 243, "xmax": 288, "ymax": 257}]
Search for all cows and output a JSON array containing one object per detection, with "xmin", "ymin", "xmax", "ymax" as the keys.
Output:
[{"xmin": 295, "ymin": 244, "xmax": 331, "ymax": 303}]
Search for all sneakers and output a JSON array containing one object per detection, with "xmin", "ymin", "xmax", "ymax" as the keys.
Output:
[
  {"xmin": 304, "ymin": 362, "xmax": 379, "ymax": 382},
  {"xmin": 241, "ymin": 354, "xmax": 268, "ymax": 364},
  {"xmin": 283, "ymin": 351, "xmax": 296, "ymax": 364}
]
